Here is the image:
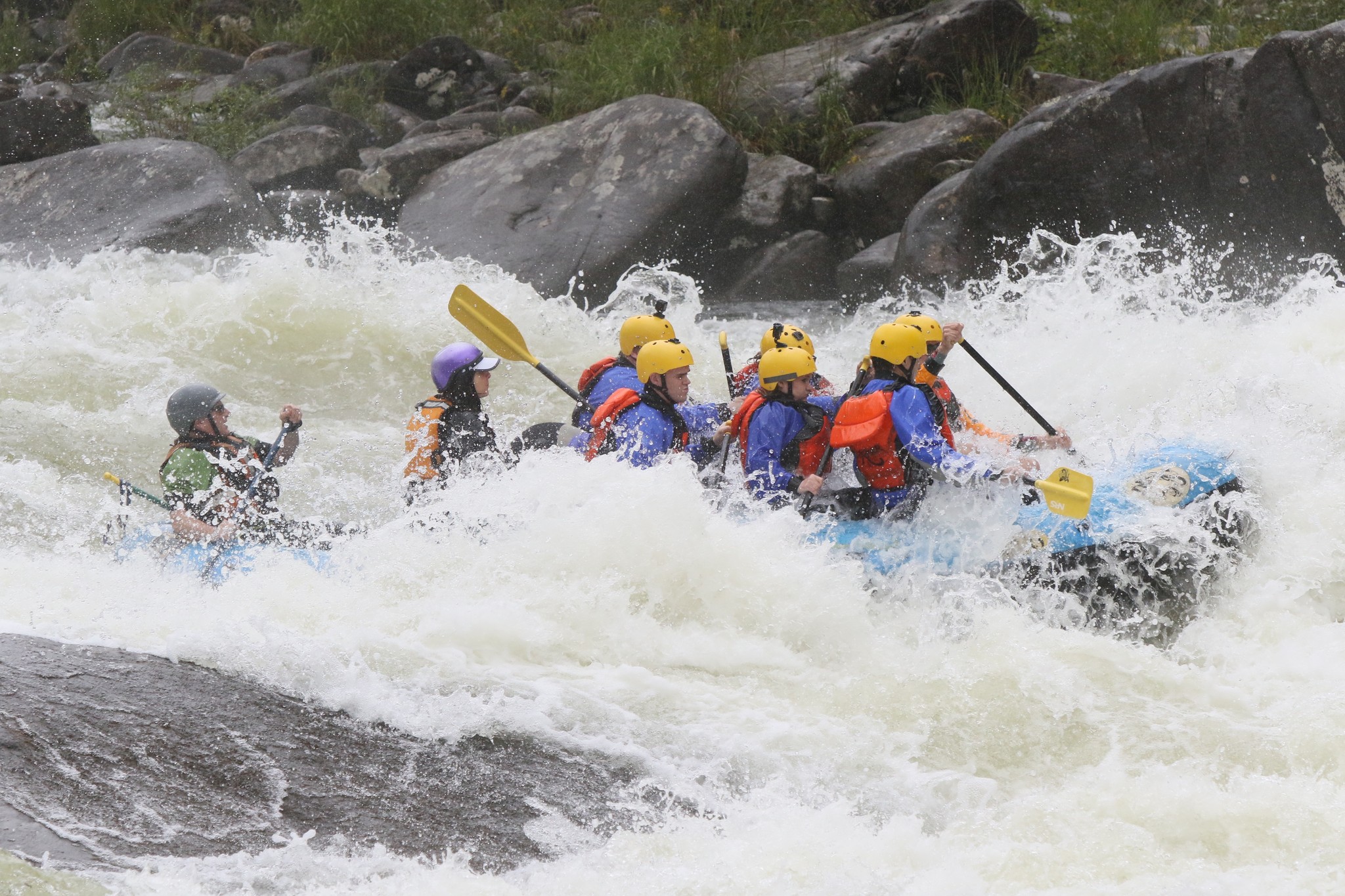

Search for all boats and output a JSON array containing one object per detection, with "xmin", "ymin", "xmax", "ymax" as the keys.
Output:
[{"xmin": 114, "ymin": 443, "xmax": 1250, "ymax": 652}]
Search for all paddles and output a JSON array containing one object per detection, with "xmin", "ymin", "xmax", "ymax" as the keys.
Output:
[
  {"xmin": 1013, "ymin": 465, "xmax": 1095, "ymax": 521},
  {"xmin": 798, "ymin": 352, "xmax": 871, "ymax": 519},
  {"xmin": 711, "ymin": 417, "xmax": 732, "ymax": 509},
  {"xmin": 955, "ymin": 332, "xmax": 1093, "ymax": 470},
  {"xmin": 103, "ymin": 471, "xmax": 174, "ymax": 513},
  {"xmin": 448, "ymin": 282, "xmax": 598, "ymax": 415},
  {"xmin": 200, "ymin": 416, "xmax": 305, "ymax": 580},
  {"xmin": 719, "ymin": 330, "xmax": 736, "ymax": 400}
]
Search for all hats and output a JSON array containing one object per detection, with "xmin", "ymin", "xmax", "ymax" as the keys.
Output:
[{"xmin": 462, "ymin": 357, "xmax": 501, "ymax": 372}]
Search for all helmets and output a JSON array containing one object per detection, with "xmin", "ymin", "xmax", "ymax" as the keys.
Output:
[
  {"xmin": 758, "ymin": 346, "xmax": 817, "ymax": 391},
  {"xmin": 869, "ymin": 323, "xmax": 928, "ymax": 365},
  {"xmin": 166, "ymin": 383, "xmax": 226, "ymax": 437},
  {"xmin": 636, "ymin": 339, "xmax": 694, "ymax": 383},
  {"xmin": 894, "ymin": 311, "xmax": 943, "ymax": 343},
  {"xmin": 620, "ymin": 313, "xmax": 676, "ymax": 355},
  {"xmin": 760, "ymin": 323, "xmax": 815, "ymax": 358},
  {"xmin": 431, "ymin": 342, "xmax": 483, "ymax": 393}
]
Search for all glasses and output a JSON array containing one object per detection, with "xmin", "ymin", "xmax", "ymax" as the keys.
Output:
[
  {"xmin": 210, "ymin": 404, "xmax": 224, "ymax": 416},
  {"xmin": 926, "ymin": 343, "xmax": 941, "ymax": 353}
]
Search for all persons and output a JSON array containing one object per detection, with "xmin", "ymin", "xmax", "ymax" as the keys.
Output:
[
  {"xmin": 573, "ymin": 308, "xmax": 1073, "ymax": 522},
  {"xmin": 159, "ymin": 383, "xmax": 369, "ymax": 551},
  {"xmin": 402, "ymin": 342, "xmax": 518, "ymax": 542}
]
